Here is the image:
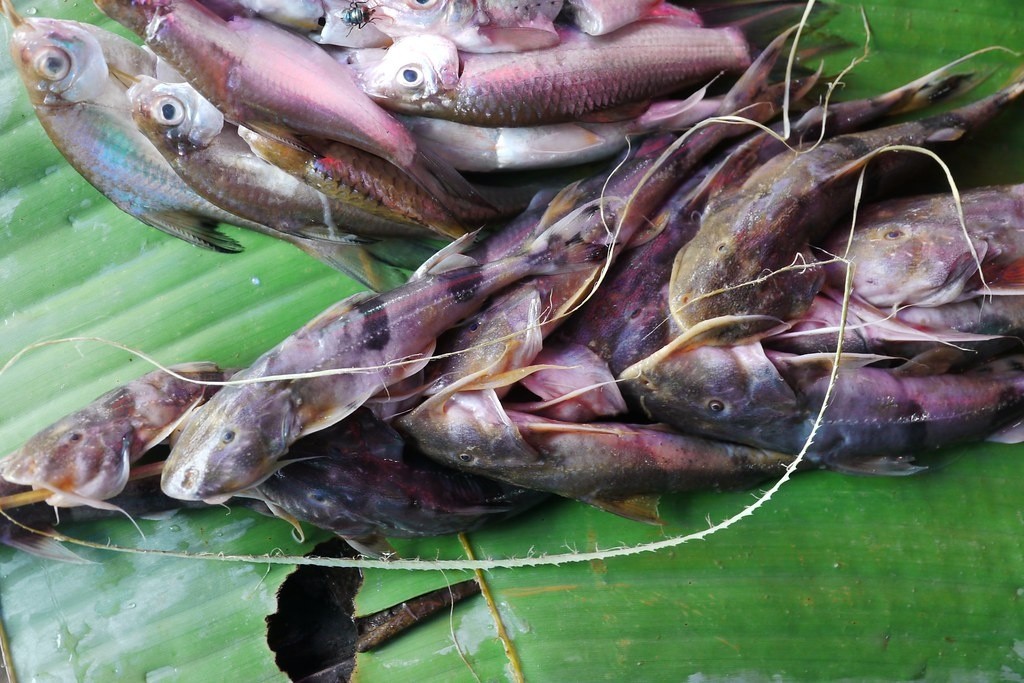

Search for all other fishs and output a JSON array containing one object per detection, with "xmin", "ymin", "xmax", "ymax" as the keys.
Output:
[{"xmin": 0, "ymin": 0, "xmax": 1024, "ymax": 561}]
